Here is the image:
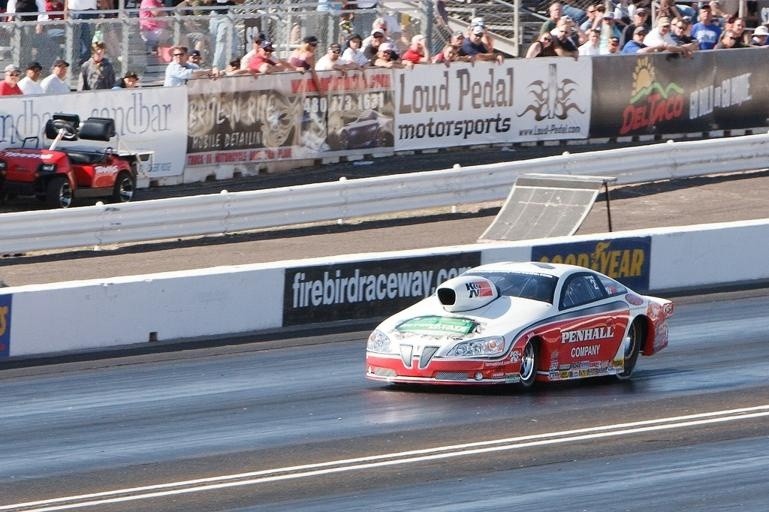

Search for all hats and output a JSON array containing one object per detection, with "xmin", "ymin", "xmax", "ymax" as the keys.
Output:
[
  {"xmin": 229, "ymin": 57, "xmax": 240, "ymax": 65},
  {"xmin": 303, "ymin": 16, "xmax": 486, "ymax": 52},
  {"xmin": 254, "ymin": 33, "xmax": 264, "ymax": 42},
  {"xmin": 588, "ymin": 4, "xmax": 768, "ymax": 43},
  {"xmin": 190, "ymin": 49, "xmax": 201, "ymax": 57},
  {"xmin": 4, "ymin": 59, "xmax": 70, "ymax": 73},
  {"xmin": 259, "ymin": 40, "xmax": 275, "ymax": 50},
  {"xmin": 124, "ymin": 71, "xmax": 140, "ymax": 80}
]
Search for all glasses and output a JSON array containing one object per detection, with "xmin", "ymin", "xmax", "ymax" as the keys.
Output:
[
  {"xmin": 264, "ymin": 48, "xmax": 272, "ymax": 52},
  {"xmin": 231, "ymin": 63, "xmax": 238, "ymax": 67},
  {"xmin": 559, "ymin": 30, "xmax": 567, "ymax": 34},
  {"xmin": 543, "ymin": 37, "xmax": 550, "ymax": 42},
  {"xmin": 10, "ymin": 73, "xmax": 18, "ymax": 76},
  {"xmin": 172, "ymin": 54, "xmax": 183, "ymax": 57}
]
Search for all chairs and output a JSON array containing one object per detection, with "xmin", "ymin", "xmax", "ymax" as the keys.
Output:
[{"xmin": 27, "ymin": 110, "xmax": 117, "ymax": 164}]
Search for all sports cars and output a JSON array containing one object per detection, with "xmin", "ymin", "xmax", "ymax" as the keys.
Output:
[
  {"xmin": 365, "ymin": 261, "xmax": 674, "ymax": 385},
  {"xmin": 336, "ymin": 108, "xmax": 393, "ymax": 147}
]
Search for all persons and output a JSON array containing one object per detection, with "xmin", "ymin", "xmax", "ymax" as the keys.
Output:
[
  {"xmin": 289, "ymin": 1, "xmax": 503, "ymax": 72},
  {"xmin": 0, "ymin": 1, "xmax": 139, "ymax": 94},
  {"xmin": 525, "ymin": 1, "xmax": 769, "ymax": 59},
  {"xmin": 139, "ymin": 0, "xmax": 305, "ymax": 86}
]
